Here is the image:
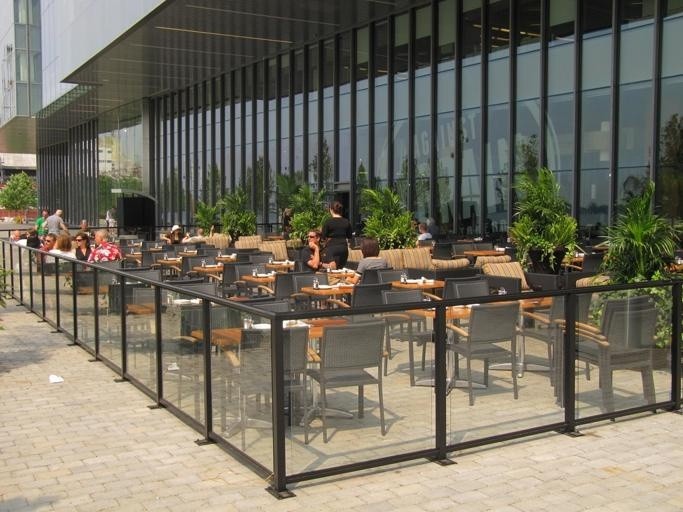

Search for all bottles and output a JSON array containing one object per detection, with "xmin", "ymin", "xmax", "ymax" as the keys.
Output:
[
  {"xmin": 130, "ymin": 247, "xmax": 134, "ymax": 255},
  {"xmin": 399, "ymin": 271, "xmax": 407, "ymax": 284},
  {"xmin": 163, "ymin": 252, "xmax": 167, "ymax": 261},
  {"xmin": 267, "ymin": 255, "xmax": 274, "ymax": 266},
  {"xmin": 251, "ymin": 267, "xmax": 258, "ymax": 279},
  {"xmin": 200, "ymin": 258, "xmax": 208, "ymax": 268},
  {"xmin": 242, "ymin": 316, "xmax": 253, "ymax": 329},
  {"xmin": 183, "ymin": 245, "xmax": 187, "ymax": 253},
  {"xmin": 165, "ymin": 293, "xmax": 175, "ymax": 305},
  {"xmin": 216, "ymin": 250, "xmax": 222, "ymax": 259},
  {"xmin": 312, "ymin": 276, "xmax": 319, "ymax": 289}
]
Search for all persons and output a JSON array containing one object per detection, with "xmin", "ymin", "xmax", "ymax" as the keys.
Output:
[
  {"xmin": 182, "ymin": 228, "xmax": 207, "ymax": 245},
  {"xmin": 300, "ymin": 228, "xmax": 336, "ymax": 272},
  {"xmin": 161, "ymin": 224, "xmax": 186, "ymax": 244},
  {"xmin": 412, "ymin": 222, "xmax": 433, "ymax": 247},
  {"xmin": 425, "ymin": 217, "xmax": 440, "ymax": 240},
  {"xmin": 343, "ymin": 233, "xmax": 388, "ymax": 285},
  {"xmin": 320, "ymin": 200, "xmax": 352, "ymax": 269},
  {"xmin": 11, "ymin": 201, "xmax": 124, "ymax": 265}
]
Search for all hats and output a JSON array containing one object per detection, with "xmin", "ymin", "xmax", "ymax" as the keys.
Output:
[{"xmin": 170, "ymin": 224, "xmax": 181, "ymax": 234}]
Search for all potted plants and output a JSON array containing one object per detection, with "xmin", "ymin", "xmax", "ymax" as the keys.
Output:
[
  {"xmin": 506, "ymin": 164, "xmax": 578, "ymax": 274},
  {"xmin": 591, "ymin": 180, "xmax": 683, "ymax": 371}
]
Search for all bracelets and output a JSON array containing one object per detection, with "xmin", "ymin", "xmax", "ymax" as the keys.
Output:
[{"xmin": 320, "ymin": 261, "xmax": 324, "ymax": 267}]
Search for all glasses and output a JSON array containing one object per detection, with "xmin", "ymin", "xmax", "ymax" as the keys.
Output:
[
  {"xmin": 305, "ymin": 235, "xmax": 314, "ymax": 238},
  {"xmin": 44, "ymin": 240, "xmax": 50, "ymax": 242},
  {"xmin": 75, "ymin": 239, "xmax": 83, "ymax": 241}
]
